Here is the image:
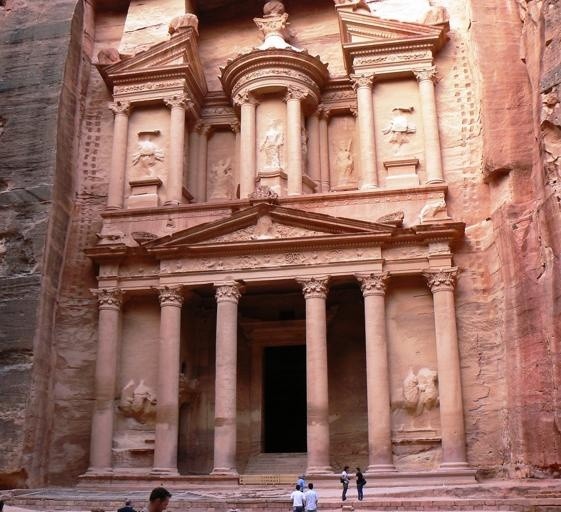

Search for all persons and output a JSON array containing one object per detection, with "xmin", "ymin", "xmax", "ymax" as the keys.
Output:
[
  {"xmin": 341, "ymin": 466, "xmax": 353, "ymax": 502},
  {"xmin": 259, "ymin": 119, "xmax": 284, "ymax": 173},
  {"xmin": 335, "ymin": 141, "xmax": 355, "ymax": 177},
  {"xmin": 351, "ymin": 468, "xmax": 363, "ymax": 501},
  {"xmin": 210, "ymin": 157, "xmax": 231, "ymax": 183},
  {"xmin": 290, "ymin": 473, "xmax": 319, "ymax": 512},
  {"xmin": 136, "ymin": 487, "xmax": 172, "ymax": 512}
]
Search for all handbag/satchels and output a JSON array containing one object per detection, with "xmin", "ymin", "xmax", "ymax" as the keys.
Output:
[{"xmin": 360, "ymin": 478, "xmax": 366, "ymax": 485}]
